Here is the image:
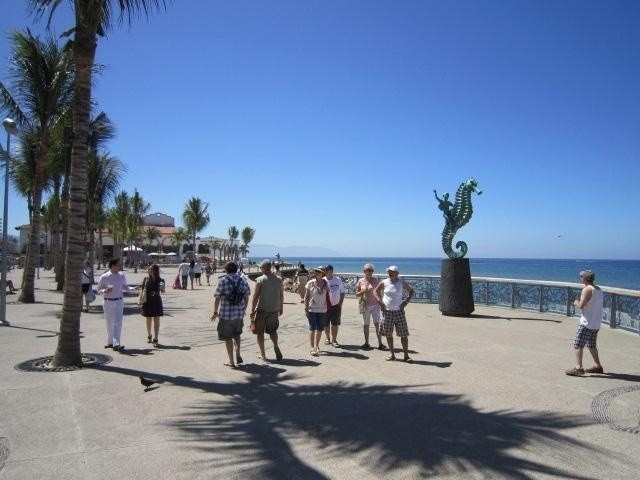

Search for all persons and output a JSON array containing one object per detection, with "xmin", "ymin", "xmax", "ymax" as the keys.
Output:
[
  {"xmin": 566, "ymin": 269, "xmax": 603, "ymax": 375},
  {"xmin": 0, "ymin": 271, "xmax": 18, "ymax": 294}
]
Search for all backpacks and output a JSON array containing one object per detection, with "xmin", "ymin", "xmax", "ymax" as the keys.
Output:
[{"xmin": 223, "ymin": 276, "xmax": 243, "ymax": 304}]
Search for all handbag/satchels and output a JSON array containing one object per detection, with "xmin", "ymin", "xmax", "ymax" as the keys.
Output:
[
  {"xmin": 326, "ymin": 292, "xmax": 331, "ymax": 311},
  {"xmin": 137, "ymin": 288, "xmax": 147, "ymax": 306},
  {"xmin": 86, "ymin": 290, "xmax": 96, "ymax": 301},
  {"xmin": 359, "ymin": 298, "xmax": 366, "ymax": 314}
]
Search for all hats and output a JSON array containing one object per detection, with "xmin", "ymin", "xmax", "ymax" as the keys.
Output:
[
  {"xmin": 314, "ymin": 266, "xmax": 327, "ymax": 275},
  {"xmin": 580, "ymin": 270, "xmax": 594, "ymax": 280},
  {"xmin": 325, "ymin": 265, "xmax": 333, "ymax": 270},
  {"xmin": 386, "ymin": 266, "xmax": 399, "ymax": 272}
]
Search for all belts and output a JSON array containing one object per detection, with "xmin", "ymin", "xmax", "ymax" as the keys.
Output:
[{"xmin": 104, "ymin": 298, "xmax": 122, "ymax": 300}]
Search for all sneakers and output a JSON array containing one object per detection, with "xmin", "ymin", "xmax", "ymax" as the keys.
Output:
[
  {"xmin": 148, "ymin": 335, "xmax": 152, "ymax": 342},
  {"xmin": 565, "ymin": 367, "xmax": 584, "ymax": 375},
  {"xmin": 153, "ymin": 339, "xmax": 158, "ymax": 347},
  {"xmin": 309, "ymin": 339, "xmax": 340, "ymax": 356},
  {"xmin": 105, "ymin": 344, "xmax": 113, "ymax": 348},
  {"xmin": 385, "ymin": 355, "xmax": 413, "ymax": 363},
  {"xmin": 274, "ymin": 346, "xmax": 282, "ymax": 360},
  {"xmin": 113, "ymin": 345, "xmax": 124, "ymax": 351},
  {"xmin": 360, "ymin": 341, "xmax": 389, "ymax": 351},
  {"xmin": 584, "ymin": 366, "xmax": 603, "ymax": 373}
]
